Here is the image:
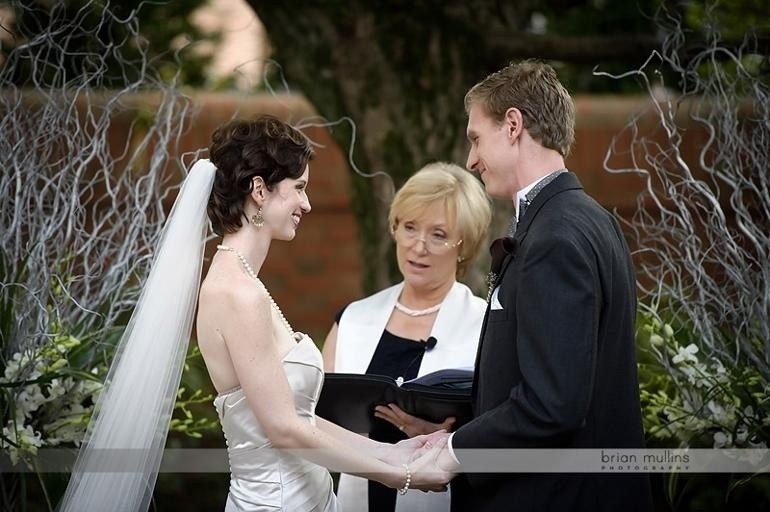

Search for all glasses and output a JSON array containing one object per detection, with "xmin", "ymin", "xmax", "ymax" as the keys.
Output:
[{"xmin": 395, "ymin": 229, "xmax": 462, "ymax": 255}]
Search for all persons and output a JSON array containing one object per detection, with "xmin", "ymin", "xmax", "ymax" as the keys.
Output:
[
  {"xmin": 313, "ymin": 161, "xmax": 493, "ymax": 512},
  {"xmin": 194, "ymin": 112, "xmax": 459, "ymax": 511},
  {"xmin": 373, "ymin": 61, "xmax": 659, "ymax": 511}
]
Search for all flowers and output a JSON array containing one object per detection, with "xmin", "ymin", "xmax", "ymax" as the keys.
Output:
[
  {"xmin": 2, "ymin": 339, "xmax": 114, "ymax": 474},
  {"xmin": 640, "ymin": 314, "xmax": 768, "ymax": 462}
]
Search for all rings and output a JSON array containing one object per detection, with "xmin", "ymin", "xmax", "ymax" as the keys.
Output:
[
  {"xmin": 399, "ymin": 425, "xmax": 405, "ymax": 431},
  {"xmin": 426, "ymin": 440, "xmax": 431, "ymax": 446}
]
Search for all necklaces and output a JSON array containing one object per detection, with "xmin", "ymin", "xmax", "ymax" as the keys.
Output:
[
  {"xmin": 215, "ymin": 243, "xmax": 298, "ymax": 343},
  {"xmin": 393, "ymin": 298, "xmax": 446, "ymax": 317}
]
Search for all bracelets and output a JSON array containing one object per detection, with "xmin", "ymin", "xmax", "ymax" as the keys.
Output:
[{"xmin": 396, "ymin": 463, "xmax": 412, "ymax": 495}]
